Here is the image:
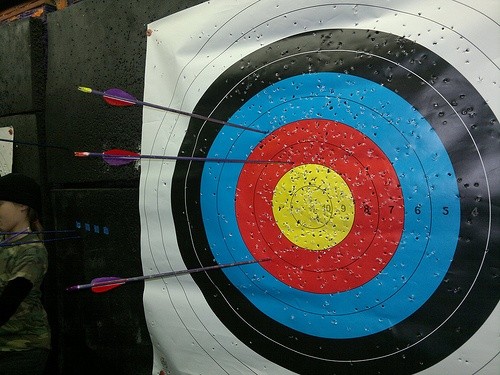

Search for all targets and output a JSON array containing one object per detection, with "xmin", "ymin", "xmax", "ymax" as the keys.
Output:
[{"xmin": 139, "ymin": 0, "xmax": 499, "ymax": 375}]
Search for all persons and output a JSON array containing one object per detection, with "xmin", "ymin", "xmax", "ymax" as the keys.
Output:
[{"xmin": 0, "ymin": 173, "xmax": 52, "ymax": 375}]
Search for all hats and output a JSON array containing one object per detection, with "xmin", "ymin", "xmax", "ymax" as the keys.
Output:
[{"xmin": 0, "ymin": 173, "xmax": 36, "ymax": 211}]
